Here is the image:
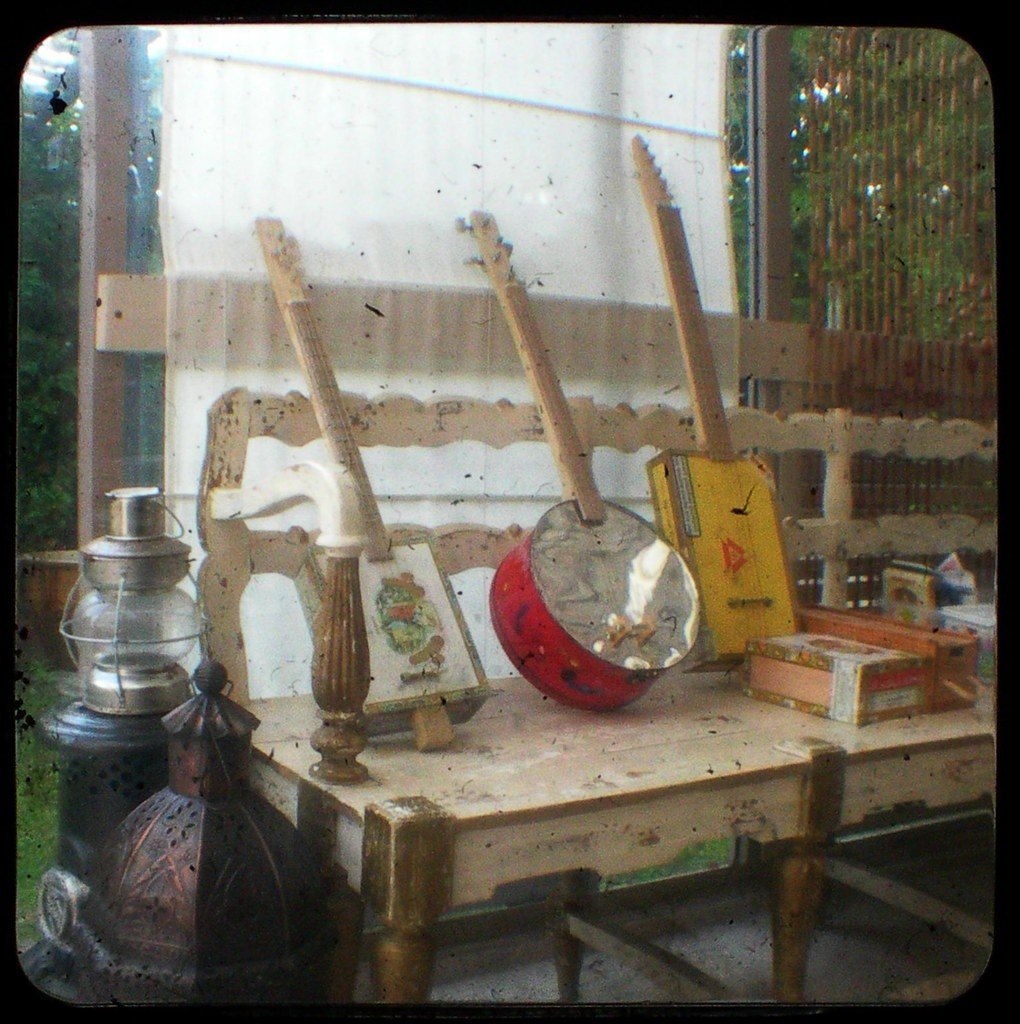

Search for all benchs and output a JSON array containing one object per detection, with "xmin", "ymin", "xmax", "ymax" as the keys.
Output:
[{"xmin": 195, "ymin": 385, "xmax": 1000, "ymax": 1005}]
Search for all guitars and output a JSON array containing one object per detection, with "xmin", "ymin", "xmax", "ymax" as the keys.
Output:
[{"xmin": 456, "ymin": 209, "xmax": 703, "ymax": 710}]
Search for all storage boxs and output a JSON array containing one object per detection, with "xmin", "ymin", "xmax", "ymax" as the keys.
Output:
[{"xmin": 741, "ymin": 558, "xmax": 997, "ymax": 725}]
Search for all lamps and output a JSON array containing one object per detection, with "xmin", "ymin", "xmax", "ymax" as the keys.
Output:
[{"xmin": 58, "ymin": 485, "xmax": 213, "ymax": 717}]
